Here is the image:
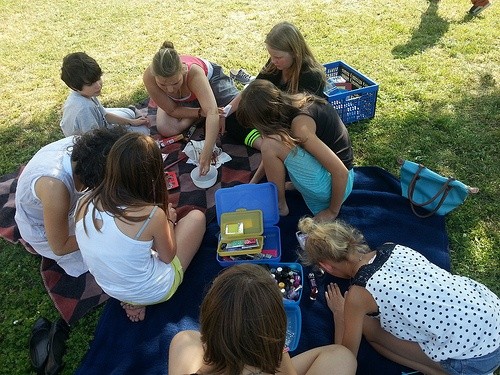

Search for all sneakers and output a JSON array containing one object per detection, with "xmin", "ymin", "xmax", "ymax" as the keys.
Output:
[
  {"xmin": 469, "ymin": 2, "xmax": 491, "ymax": 16},
  {"xmin": 230, "ymin": 70, "xmax": 256, "ymax": 84}
]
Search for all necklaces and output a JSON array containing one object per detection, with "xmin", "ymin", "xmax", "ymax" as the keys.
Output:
[{"xmin": 350, "ymin": 255, "xmax": 363, "ymax": 279}]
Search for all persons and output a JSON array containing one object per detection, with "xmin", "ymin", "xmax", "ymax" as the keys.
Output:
[
  {"xmin": 59, "ymin": 52, "xmax": 150, "ymax": 138},
  {"xmin": 73, "ymin": 133, "xmax": 206, "ymax": 322},
  {"xmin": 298, "ymin": 217, "xmax": 500, "ymax": 375},
  {"xmin": 143, "ymin": 41, "xmax": 240, "ymax": 177},
  {"xmin": 239, "ymin": 79, "xmax": 354, "ymax": 225},
  {"xmin": 469, "ymin": 0, "xmax": 492, "ymax": 16},
  {"xmin": 217, "ymin": 22, "xmax": 327, "ymax": 151},
  {"xmin": 168, "ymin": 263, "xmax": 357, "ymax": 375},
  {"xmin": 14, "ymin": 121, "xmax": 130, "ymax": 278}
]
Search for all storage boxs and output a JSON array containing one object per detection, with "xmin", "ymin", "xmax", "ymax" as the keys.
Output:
[
  {"xmin": 262, "ymin": 260, "xmax": 303, "ymax": 352},
  {"xmin": 213, "ymin": 180, "xmax": 283, "ymax": 264},
  {"xmin": 320, "ymin": 60, "xmax": 380, "ymax": 126}
]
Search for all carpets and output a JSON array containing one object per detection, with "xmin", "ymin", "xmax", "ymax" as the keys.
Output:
[{"xmin": 0, "ymin": 96, "xmax": 452, "ymax": 375}]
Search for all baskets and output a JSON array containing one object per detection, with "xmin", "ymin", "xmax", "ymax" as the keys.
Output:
[{"xmin": 320, "ymin": 61, "xmax": 379, "ymax": 125}]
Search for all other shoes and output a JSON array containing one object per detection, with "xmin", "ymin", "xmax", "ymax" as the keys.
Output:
[
  {"xmin": 29, "ymin": 317, "xmax": 51, "ymax": 373},
  {"xmin": 36, "ymin": 319, "xmax": 68, "ymax": 375}
]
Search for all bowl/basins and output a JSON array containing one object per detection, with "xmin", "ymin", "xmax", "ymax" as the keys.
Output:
[{"xmin": 190, "ymin": 166, "xmax": 218, "ymax": 188}]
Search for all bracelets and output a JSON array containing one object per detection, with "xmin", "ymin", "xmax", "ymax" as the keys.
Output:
[
  {"xmin": 283, "ymin": 347, "xmax": 290, "ymax": 353},
  {"xmin": 198, "ymin": 107, "xmax": 203, "ymax": 119},
  {"xmin": 168, "ymin": 218, "xmax": 175, "ymax": 226}
]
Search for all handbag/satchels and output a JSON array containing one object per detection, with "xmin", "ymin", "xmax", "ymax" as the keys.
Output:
[{"xmin": 401, "ymin": 161, "xmax": 470, "ymax": 217}]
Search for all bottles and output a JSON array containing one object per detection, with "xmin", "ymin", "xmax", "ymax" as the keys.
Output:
[
  {"xmin": 269, "ymin": 266, "xmax": 302, "ymax": 301},
  {"xmin": 310, "ymin": 288, "xmax": 319, "ymax": 300}
]
